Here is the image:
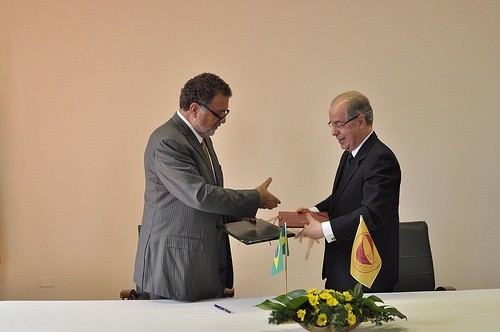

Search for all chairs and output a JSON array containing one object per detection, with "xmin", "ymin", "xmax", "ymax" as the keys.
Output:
[
  {"xmin": 120, "ymin": 225, "xmax": 236, "ymax": 299},
  {"xmin": 396, "ymin": 221, "xmax": 457, "ymax": 292}
]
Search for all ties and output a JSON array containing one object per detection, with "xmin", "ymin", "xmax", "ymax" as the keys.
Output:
[
  {"xmin": 342, "ymin": 152, "xmax": 352, "ymax": 176},
  {"xmin": 201, "ymin": 140, "xmax": 214, "ymax": 175}
]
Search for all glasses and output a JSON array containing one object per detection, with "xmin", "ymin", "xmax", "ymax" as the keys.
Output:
[
  {"xmin": 194, "ymin": 102, "xmax": 230, "ymax": 123},
  {"xmin": 327, "ymin": 114, "xmax": 362, "ymax": 129}
]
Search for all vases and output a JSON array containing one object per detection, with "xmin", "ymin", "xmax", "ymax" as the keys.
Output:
[{"xmin": 301, "ymin": 320, "xmax": 363, "ymax": 332}]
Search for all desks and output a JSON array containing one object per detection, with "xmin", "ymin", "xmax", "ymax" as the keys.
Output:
[{"xmin": 0, "ymin": 282, "xmax": 500, "ymax": 332}]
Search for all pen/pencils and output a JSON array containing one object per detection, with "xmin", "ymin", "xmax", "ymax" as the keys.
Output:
[{"xmin": 214, "ymin": 304, "xmax": 231, "ymax": 313}]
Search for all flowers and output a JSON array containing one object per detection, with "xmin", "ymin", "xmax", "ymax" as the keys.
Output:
[{"xmin": 258, "ymin": 283, "xmax": 410, "ymax": 325}]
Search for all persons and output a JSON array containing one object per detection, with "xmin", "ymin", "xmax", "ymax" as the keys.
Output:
[
  {"xmin": 133, "ymin": 72, "xmax": 281, "ymax": 302},
  {"xmin": 296, "ymin": 90, "xmax": 401, "ymax": 293}
]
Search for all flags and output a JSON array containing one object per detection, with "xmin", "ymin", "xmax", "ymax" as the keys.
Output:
[
  {"xmin": 350, "ymin": 218, "xmax": 382, "ymax": 288},
  {"xmin": 271, "ymin": 224, "xmax": 289, "ymax": 275}
]
